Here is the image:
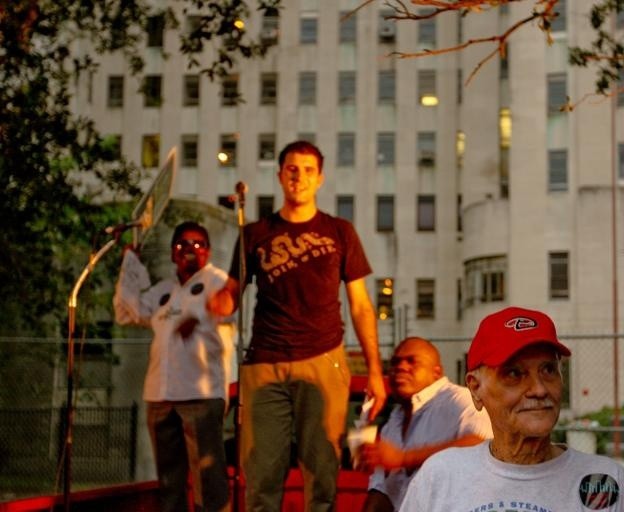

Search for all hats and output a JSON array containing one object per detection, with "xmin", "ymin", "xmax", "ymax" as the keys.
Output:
[{"xmin": 465, "ymin": 306, "xmax": 572, "ymax": 372}]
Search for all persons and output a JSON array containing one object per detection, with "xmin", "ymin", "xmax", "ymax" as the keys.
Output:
[
  {"xmin": 398, "ymin": 305, "xmax": 624, "ymax": 511},
  {"xmin": 114, "ymin": 217, "xmax": 237, "ymax": 511},
  {"xmin": 363, "ymin": 334, "xmax": 495, "ymax": 511},
  {"xmin": 205, "ymin": 138, "xmax": 389, "ymax": 511}
]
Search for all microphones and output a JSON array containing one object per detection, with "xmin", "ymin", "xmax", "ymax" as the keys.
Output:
[
  {"xmin": 106, "ymin": 218, "xmax": 145, "ymax": 234},
  {"xmin": 235, "ymin": 182, "xmax": 246, "ymax": 205}
]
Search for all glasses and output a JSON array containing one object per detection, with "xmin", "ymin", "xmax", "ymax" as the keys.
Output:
[{"xmin": 174, "ymin": 238, "xmax": 210, "ymax": 251}]
